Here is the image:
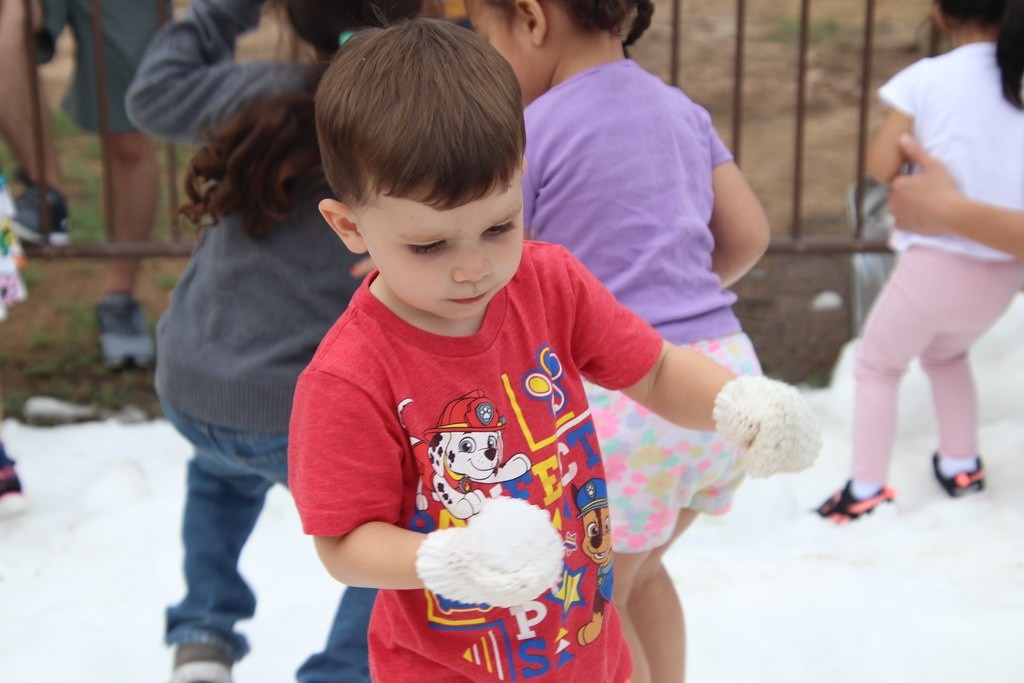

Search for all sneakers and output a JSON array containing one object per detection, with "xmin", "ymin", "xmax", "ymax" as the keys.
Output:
[
  {"xmin": 9, "ymin": 187, "xmax": 71, "ymax": 246},
  {"xmin": 96, "ymin": 291, "xmax": 154, "ymax": 368},
  {"xmin": 933, "ymin": 454, "xmax": 986, "ymax": 498},
  {"xmin": 817, "ymin": 481, "xmax": 894, "ymax": 524}
]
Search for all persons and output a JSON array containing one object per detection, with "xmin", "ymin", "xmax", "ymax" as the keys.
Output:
[
  {"xmin": 0, "ymin": 172, "xmax": 24, "ymax": 514},
  {"xmin": 126, "ymin": 0, "xmax": 431, "ymax": 683},
  {"xmin": 1, "ymin": 0, "xmax": 170, "ymax": 370},
  {"xmin": 8, "ymin": 166, "xmax": 69, "ymax": 246},
  {"xmin": 460, "ymin": 0, "xmax": 765, "ymax": 683},
  {"xmin": 288, "ymin": 19, "xmax": 816, "ymax": 683},
  {"xmin": 887, "ymin": 132, "xmax": 1024, "ymax": 263},
  {"xmin": 818, "ymin": 2, "xmax": 1024, "ymax": 525}
]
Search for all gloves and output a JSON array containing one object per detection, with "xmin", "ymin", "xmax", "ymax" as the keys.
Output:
[
  {"xmin": 713, "ymin": 374, "xmax": 823, "ymax": 480},
  {"xmin": 419, "ymin": 493, "xmax": 566, "ymax": 609}
]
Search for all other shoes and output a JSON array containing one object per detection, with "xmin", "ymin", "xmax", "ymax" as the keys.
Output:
[
  {"xmin": 172, "ymin": 642, "xmax": 233, "ymax": 683},
  {"xmin": 0, "ymin": 461, "xmax": 23, "ymax": 516}
]
